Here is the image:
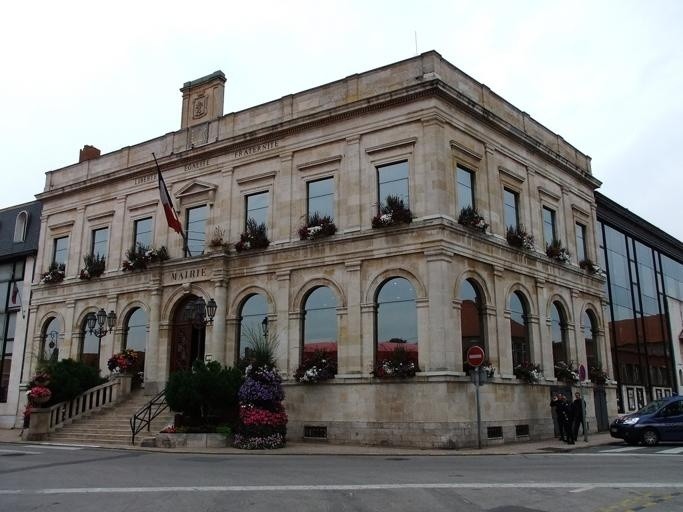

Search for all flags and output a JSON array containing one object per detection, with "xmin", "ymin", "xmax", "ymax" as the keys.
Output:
[{"xmin": 158, "ymin": 168, "xmax": 181, "ymax": 235}]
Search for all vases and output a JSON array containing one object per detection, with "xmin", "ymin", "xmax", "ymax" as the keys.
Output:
[{"xmin": 31, "ymin": 396, "xmax": 50, "ymax": 408}]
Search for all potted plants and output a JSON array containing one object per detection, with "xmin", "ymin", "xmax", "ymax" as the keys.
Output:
[{"xmin": 159, "ymin": 359, "xmax": 246, "ymax": 449}]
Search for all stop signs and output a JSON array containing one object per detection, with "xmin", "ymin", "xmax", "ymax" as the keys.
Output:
[{"xmin": 467, "ymin": 346, "xmax": 484, "ymax": 367}]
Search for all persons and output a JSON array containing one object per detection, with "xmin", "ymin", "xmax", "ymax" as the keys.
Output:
[{"xmin": 550, "ymin": 392, "xmax": 586, "ymax": 445}]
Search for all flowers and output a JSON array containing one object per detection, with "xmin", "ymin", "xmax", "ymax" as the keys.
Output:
[
  {"xmin": 588, "ymin": 362, "xmax": 609, "ymax": 386},
  {"xmin": 370, "ymin": 344, "xmax": 418, "ymax": 381},
  {"xmin": 464, "ymin": 361, "xmax": 497, "ymax": 378},
  {"xmin": 294, "ymin": 344, "xmax": 334, "ymax": 386},
  {"xmin": 25, "ymin": 387, "xmax": 51, "ymax": 402},
  {"xmin": 40, "ymin": 261, "xmax": 66, "ymax": 284},
  {"xmin": 107, "ymin": 348, "xmax": 141, "ymax": 372},
  {"xmin": 234, "ymin": 217, "xmax": 271, "ymax": 256},
  {"xmin": 121, "ymin": 242, "xmax": 169, "ymax": 274},
  {"xmin": 79, "ymin": 252, "xmax": 107, "ymax": 279},
  {"xmin": 204, "ymin": 225, "xmax": 229, "ymax": 254},
  {"xmin": 579, "ymin": 257, "xmax": 600, "ymax": 275},
  {"xmin": 505, "ymin": 223, "xmax": 535, "ymax": 251},
  {"xmin": 457, "ymin": 204, "xmax": 489, "ymax": 235},
  {"xmin": 513, "ymin": 361, "xmax": 543, "ymax": 384},
  {"xmin": 231, "ymin": 320, "xmax": 288, "ymax": 450},
  {"xmin": 546, "ymin": 237, "xmax": 570, "ymax": 265},
  {"xmin": 370, "ymin": 195, "xmax": 414, "ymax": 230},
  {"xmin": 555, "ymin": 359, "xmax": 582, "ymax": 383},
  {"xmin": 298, "ymin": 209, "xmax": 337, "ymax": 243}
]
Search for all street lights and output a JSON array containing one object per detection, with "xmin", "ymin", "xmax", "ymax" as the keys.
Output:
[
  {"xmin": 86, "ymin": 306, "xmax": 119, "ymax": 383},
  {"xmin": 182, "ymin": 295, "xmax": 218, "ymax": 373}
]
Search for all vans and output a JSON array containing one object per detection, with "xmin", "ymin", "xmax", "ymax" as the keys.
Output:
[{"xmin": 609, "ymin": 394, "xmax": 682, "ymax": 446}]
